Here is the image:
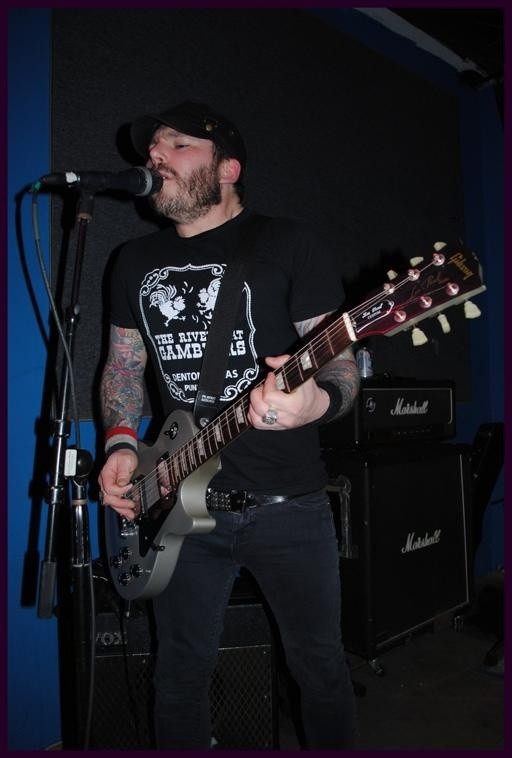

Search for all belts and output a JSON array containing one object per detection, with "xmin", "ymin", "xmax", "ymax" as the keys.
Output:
[{"xmin": 203, "ymin": 486, "xmax": 326, "ymax": 515}]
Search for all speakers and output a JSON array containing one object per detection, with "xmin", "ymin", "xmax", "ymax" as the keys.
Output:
[
  {"xmin": 323, "ymin": 438, "xmax": 472, "ymax": 652},
  {"xmin": 61, "ymin": 599, "xmax": 277, "ymax": 758}
]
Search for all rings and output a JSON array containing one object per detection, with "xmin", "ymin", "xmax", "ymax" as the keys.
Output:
[{"xmin": 261, "ymin": 416, "xmax": 276, "ymax": 424}]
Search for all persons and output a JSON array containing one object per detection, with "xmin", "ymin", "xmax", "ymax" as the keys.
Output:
[{"xmin": 87, "ymin": 97, "xmax": 363, "ymax": 750}]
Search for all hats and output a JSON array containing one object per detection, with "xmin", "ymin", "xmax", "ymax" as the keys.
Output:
[{"xmin": 110, "ymin": 98, "xmax": 249, "ymax": 171}]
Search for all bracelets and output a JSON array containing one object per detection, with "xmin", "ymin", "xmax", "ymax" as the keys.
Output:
[
  {"xmin": 103, "ymin": 426, "xmax": 140, "ymax": 458},
  {"xmin": 308, "ymin": 380, "xmax": 342, "ymax": 432}
]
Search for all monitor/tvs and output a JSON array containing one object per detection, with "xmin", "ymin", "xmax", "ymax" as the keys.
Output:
[{"xmin": 42, "ymin": 165, "xmax": 164, "ymax": 197}]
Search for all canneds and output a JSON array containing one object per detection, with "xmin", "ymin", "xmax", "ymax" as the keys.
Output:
[{"xmin": 356, "ymin": 345, "xmax": 374, "ymax": 378}]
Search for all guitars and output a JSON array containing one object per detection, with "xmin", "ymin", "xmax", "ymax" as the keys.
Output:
[{"xmin": 104, "ymin": 239, "xmax": 488, "ymax": 599}]
